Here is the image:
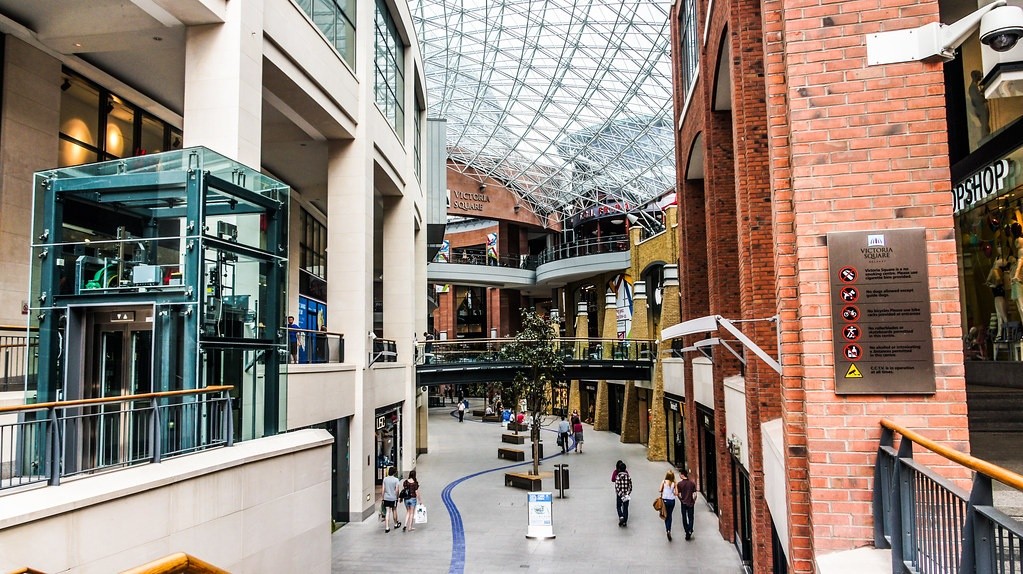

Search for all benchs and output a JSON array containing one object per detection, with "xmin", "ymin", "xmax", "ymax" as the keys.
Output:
[
  {"xmin": 473, "ymin": 410, "xmax": 485, "ymax": 416},
  {"xmin": 482, "ymin": 415, "xmax": 503, "ymax": 422},
  {"xmin": 497, "ymin": 448, "xmax": 524, "ymax": 462},
  {"xmin": 502, "ymin": 433, "xmax": 524, "ymax": 445},
  {"xmin": 508, "ymin": 423, "xmax": 527, "ymax": 431},
  {"xmin": 505, "ymin": 472, "xmax": 542, "ymax": 492}
]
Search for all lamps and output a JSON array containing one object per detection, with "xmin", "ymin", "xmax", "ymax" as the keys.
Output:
[
  {"xmin": 641, "ymin": 350, "xmax": 659, "ymax": 363},
  {"xmin": 61, "ymin": 77, "xmax": 72, "ymax": 92},
  {"xmin": 106, "ymin": 101, "xmax": 114, "ymax": 114},
  {"xmin": 681, "ymin": 345, "xmax": 715, "ymax": 367},
  {"xmin": 610, "ymin": 213, "xmax": 639, "ymax": 225},
  {"xmin": 660, "ymin": 313, "xmax": 782, "ymax": 377},
  {"xmin": 693, "ymin": 336, "xmax": 746, "ymax": 368},
  {"xmin": 615, "ymin": 352, "xmax": 632, "ymax": 362},
  {"xmin": 172, "ymin": 137, "xmax": 180, "ymax": 147},
  {"xmin": 592, "ymin": 230, "xmax": 604, "ymax": 236},
  {"xmin": 660, "ymin": 348, "xmax": 686, "ymax": 362}
]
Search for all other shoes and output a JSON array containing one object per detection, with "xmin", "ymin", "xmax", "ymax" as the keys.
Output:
[
  {"xmin": 686, "ymin": 529, "xmax": 691, "ymax": 540},
  {"xmin": 394, "ymin": 523, "xmax": 401, "ymax": 528},
  {"xmin": 667, "ymin": 530, "xmax": 671, "ymax": 542},
  {"xmin": 408, "ymin": 527, "xmax": 415, "ymax": 531},
  {"xmin": 403, "ymin": 526, "xmax": 406, "ymax": 531},
  {"xmin": 619, "ymin": 517, "xmax": 627, "ymax": 527},
  {"xmin": 385, "ymin": 528, "xmax": 390, "ymax": 533}
]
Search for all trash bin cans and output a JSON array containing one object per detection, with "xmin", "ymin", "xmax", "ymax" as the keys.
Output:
[
  {"xmin": 532, "ymin": 440, "xmax": 543, "ymax": 465},
  {"xmin": 553, "ymin": 464, "xmax": 569, "ymax": 499}
]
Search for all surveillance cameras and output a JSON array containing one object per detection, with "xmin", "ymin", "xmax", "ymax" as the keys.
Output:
[{"xmin": 979, "ymin": 6, "xmax": 1023, "ymax": 53}]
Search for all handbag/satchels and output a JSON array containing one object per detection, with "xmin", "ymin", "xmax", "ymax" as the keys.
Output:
[
  {"xmin": 653, "ymin": 498, "xmax": 662, "ymax": 510},
  {"xmin": 414, "ymin": 504, "xmax": 427, "ymax": 523},
  {"xmin": 399, "ymin": 488, "xmax": 410, "ymax": 499},
  {"xmin": 557, "ymin": 436, "xmax": 562, "ymax": 446},
  {"xmin": 380, "ymin": 502, "xmax": 387, "ymax": 521}
]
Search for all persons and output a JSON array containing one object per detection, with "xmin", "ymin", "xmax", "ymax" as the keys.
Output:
[
  {"xmin": 286, "ymin": 316, "xmax": 301, "ymax": 364},
  {"xmin": 659, "ymin": 470, "xmax": 678, "ymax": 541},
  {"xmin": 510, "ymin": 411, "xmax": 533, "ymax": 428},
  {"xmin": 985, "ymin": 223, "xmax": 1023, "ymax": 342},
  {"xmin": 611, "ymin": 460, "xmax": 632, "ymax": 527},
  {"xmin": 569, "ymin": 409, "xmax": 583, "ymax": 453},
  {"xmin": 457, "ymin": 387, "xmax": 464, "ymax": 402},
  {"xmin": 402, "ymin": 470, "xmax": 422, "ymax": 532},
  {"xmin": 382, "ymin": 467, "xmax": 401, "ymax": 533},
  {"xmin": 458, "ymin": 401, "xmax": 465, "ymax": 422},
  {"xmin": 486, "ymin": 396, "xmax": 505, "ymax": 427},
  {"xmin": 444, "ymin": 385, "xmax": 455, "ymax": 399},
  {"xmin": 677, "ymin": 468, "xmax": 697, "ymax": 540},
  {"xmin": 462, "ymin": 249, "xmax": 467, "ymax": 263},
  {"xmin": 969, "ymin": 70, "xmax": 990, "ymax": 139},
  {"xmin": 422, "ymin": 332, "xmax": 434, "ymax": 364},
  {"xmin": 558, "ymin": 416, "xmax": 570, "ymax": 453}
]
